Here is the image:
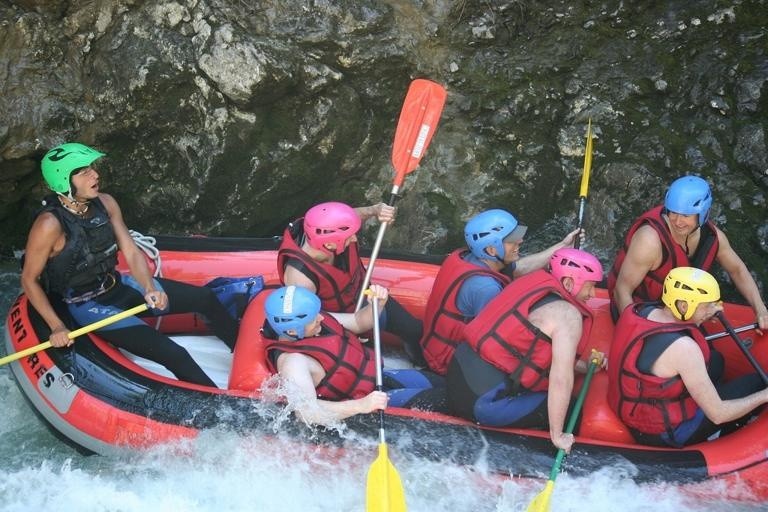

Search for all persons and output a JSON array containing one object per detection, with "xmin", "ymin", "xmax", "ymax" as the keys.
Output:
[
  {"xmin": 447, "ymin": 246, "xmax": 608, "ymax": 456},
  {"xmin": 277, "ymin": 199, "xmax": 424, "ymax": 368},
  {"xmin": 419, "ymin": 209, "xmax": 586, "ymax": 376},
  {"xmin": 264, "ymin": 283, "xmax": 454, "ymax": 426},
  {"xmin": 606, "ymin": 265, "xmax": 768, "ymax": 449},
  {"xmin": 21, "ymin": 143, "xmax": 238, "ymax": 391},
  {"xmin": 606, "ymin": 174, "xmax": 768, "ymax": 338}
]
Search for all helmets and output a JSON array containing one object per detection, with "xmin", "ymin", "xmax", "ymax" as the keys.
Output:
[
  {"xmin": 464, "ymin": 209, "xmax": 517, "ymax": 261},
  {"xmin": 304, "ymin": 201, "xmax": 362, "ymax": 256},
  {"xmin": 264, "ymin": 285, "xmax": 321, "ymax": 340},
  {"xmin": 664, "ymin": 175, "xmax": 713, "ymax": 226},
  {"xmin": 40, "ymin": 142, "xmax": 105, "ymax": 194},
  {"xmin": 662, "ymin": 266, "xmax": 720, "ymax": 319},
  {"xmin": 548, "ymin": 248, "xmax": 603, "ymax": 297}
]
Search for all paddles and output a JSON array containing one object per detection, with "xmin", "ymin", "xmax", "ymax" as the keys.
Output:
[
  {"xmin": 352, "ymin": 80, "xmax": 448, "ymax": 343},
  {"xmin": 525, "ymin": 346, "xmax": 605, "ymax": 510},
  {"xmin": 573, "ymin": 117, "xmax": 593, "ymax": 251},
  {"xmin": 366, "ymin": 287, "xmax": 406, "ymax": 512}
]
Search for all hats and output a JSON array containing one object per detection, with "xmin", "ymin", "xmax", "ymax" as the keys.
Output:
[{"xmin": 503, "ymin": 225, "xmax": 528, "ymax": 242}]
